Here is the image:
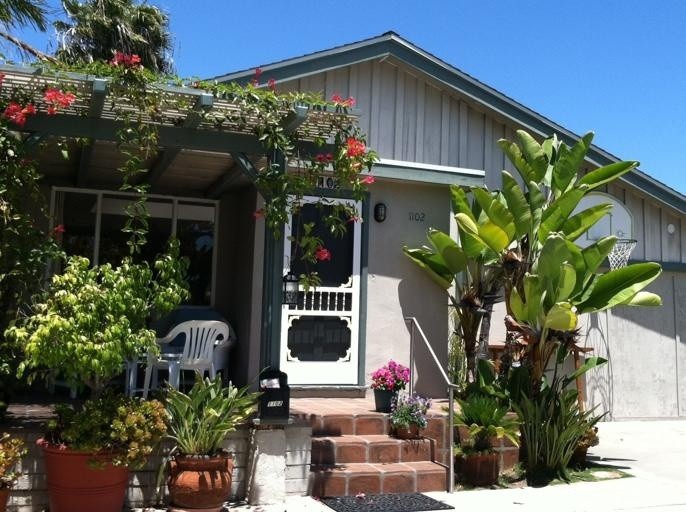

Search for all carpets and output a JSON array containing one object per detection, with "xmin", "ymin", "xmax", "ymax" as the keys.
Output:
[{"xmin": 317, "ymin": 490, "xmax": 457, "ymax": 512}]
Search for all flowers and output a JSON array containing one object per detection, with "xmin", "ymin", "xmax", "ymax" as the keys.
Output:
[
  {"xmin": 368, "ymin": 359, "xmax": 411, "ymax": 391},
  {"xmin": 389, "ymin": 390, "xmax": 433, "ymax": 435}
]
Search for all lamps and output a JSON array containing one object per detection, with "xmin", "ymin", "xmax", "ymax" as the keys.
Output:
[
  {"xmin": 374, "ymin": 203, "xmax": 386, "ymax": 223},
  {"xmin": 282, "ymin": 131, "xmax": 300, "ymax": 305}
]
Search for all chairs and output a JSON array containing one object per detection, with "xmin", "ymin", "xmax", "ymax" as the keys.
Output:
[{"xmin": 124, "ymin": 310, "xmax": 238, "ymax": 402}]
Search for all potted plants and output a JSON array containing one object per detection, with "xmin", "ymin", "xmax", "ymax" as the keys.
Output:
[
  {"xmin": 0, "ymin": 431, "xmax": 32, "ymax": 512},
  {"xmin": 153, "ymin": 366, "xmax": 266, "ymax": 512},
  {"xmin": 0, "ymin": 232, "xmax": 192, "ymax": 512},
  {"xmin": 440, "ymin": 386, "xmax": 526, "ymax": 486}
]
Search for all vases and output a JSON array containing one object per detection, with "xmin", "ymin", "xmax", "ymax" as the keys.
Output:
[
  {"xmin": 373, "ymin": 389, "xmax": 398, "ymax": 413},
  {"xmin": 395, "ymin": 420, "xmax": 418, "ymax": 439}
]
